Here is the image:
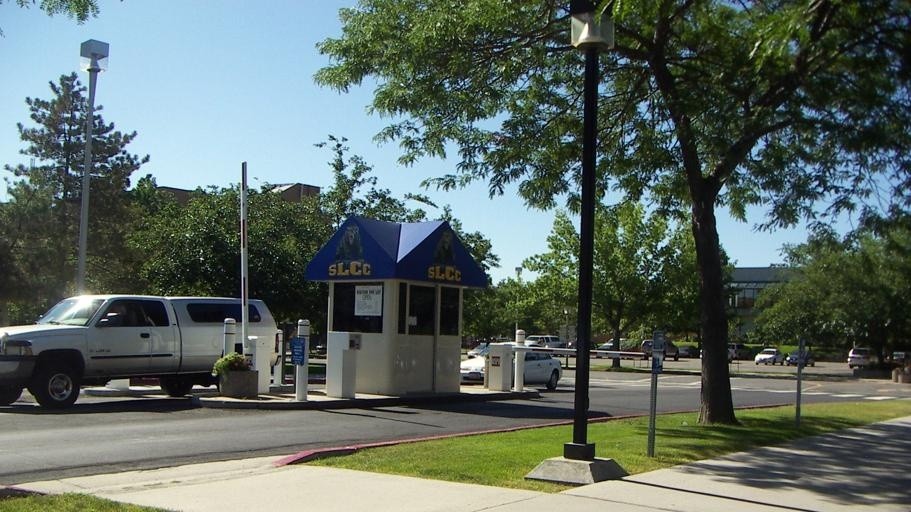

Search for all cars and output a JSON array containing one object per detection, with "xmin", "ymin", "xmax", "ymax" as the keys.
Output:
[
  {"xmin": 597, "ymin": 338, "xmax": 631, "ymax": 354},
  {"xmin": 459, "ymin": 334, "xmax": 563, "ymax": 390},
  {"xmin": 640, "ymin": 339, "xmax": 752, "ymax": 363},
  {"xmin": 755, "ymin": 348, "xmax": 784, "ymax": 365},
  {"xmin": 786, "ymin": 350, "xmax": 815, "ymax": 367},
  {"xmin": 846, "ymin": 347, "xmax": 911, "ymax": 369},
  {"xmin": 561, "ymin": 340, "xmax": 595, "ymax": 357}
]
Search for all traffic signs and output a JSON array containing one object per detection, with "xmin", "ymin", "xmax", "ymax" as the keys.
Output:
[{"xmin": 77, "ymin": 40, "xmax": 109, "ymax": 295}]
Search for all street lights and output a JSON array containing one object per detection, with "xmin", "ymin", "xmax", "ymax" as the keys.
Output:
[{"xmin": 523, "ymin": 0, "xmax": 632, "ymax": 486}]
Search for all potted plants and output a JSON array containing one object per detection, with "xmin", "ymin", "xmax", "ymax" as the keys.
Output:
[{"xmin": 211, "ymin": 352, "xmax": 258, "ymax": 399}]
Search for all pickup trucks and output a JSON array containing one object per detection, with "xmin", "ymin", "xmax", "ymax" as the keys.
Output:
[{"xmin": 0, "ymin": 294, "xmax": 282, "ymax": 408}]
